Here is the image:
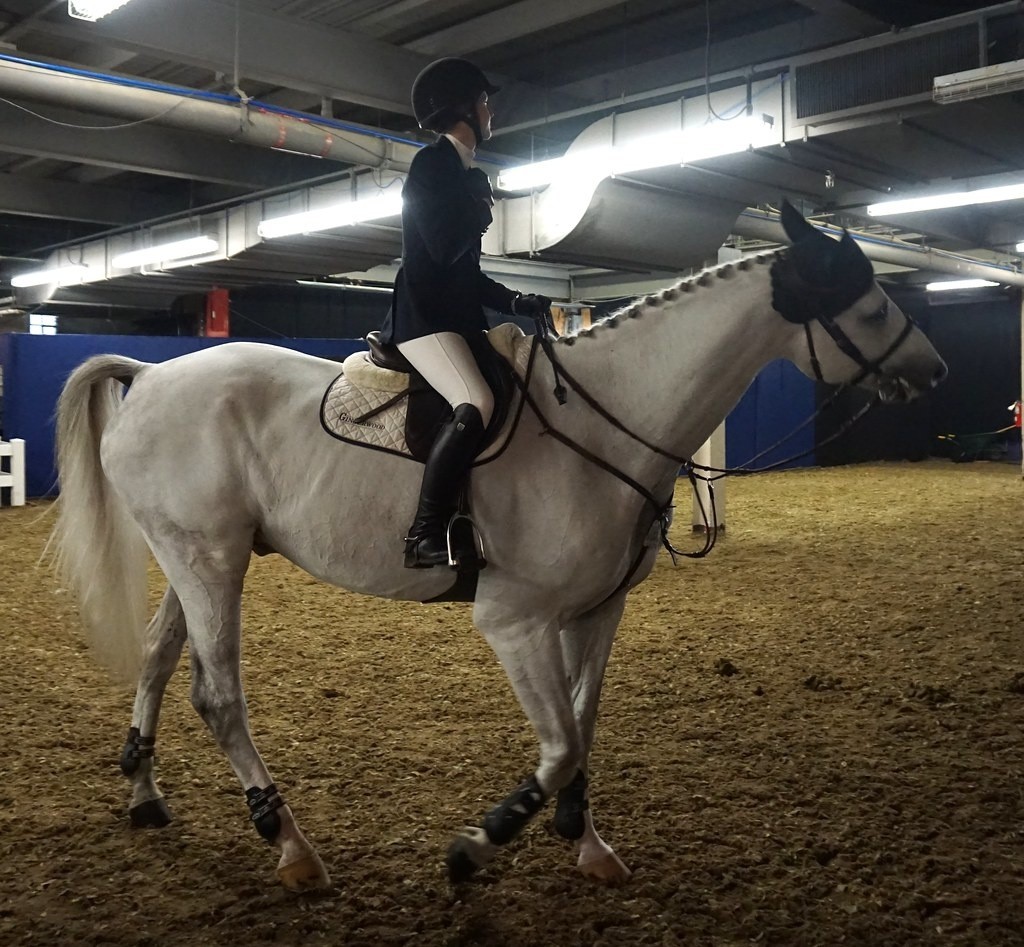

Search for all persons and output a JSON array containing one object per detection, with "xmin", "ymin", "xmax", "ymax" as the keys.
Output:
[{"xmin": 388, "ymin": 58, "xmax": 551, "ymax": 568}]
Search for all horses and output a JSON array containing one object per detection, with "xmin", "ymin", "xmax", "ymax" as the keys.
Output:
[{"xmin": 26, "ymin": 195, "xmax": 950, "ymax": 897}]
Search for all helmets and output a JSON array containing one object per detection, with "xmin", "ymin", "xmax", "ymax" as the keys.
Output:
[{"xmin": 413, "ymin": 57, "xmax": 501, "ymax": 131}]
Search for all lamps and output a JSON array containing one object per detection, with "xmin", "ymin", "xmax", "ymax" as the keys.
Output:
[
  {"xmin": 926, "ymin": 278, "xmax": 1000, "ymax": 291},
  {"xmin": 867, "ymin": 183, "xmax": 1024, "ymax": 217},
  {"xmin": 113, "ymin": 236, "xmax": 219, "ymax": 268},
  {"xmin": 68, "ymin": 0, "xmax": 132, "ymax": 24},
  {"xmin": 258, "ymin": 185, "xmax": 404, "ymax": 240},
  {"xmin": 11, "ymin": 262, "xmax": 100, "ymax": 288},
  {"xmin": 495, "ymin": 114, "xmax": 774, "ymax": 193}
]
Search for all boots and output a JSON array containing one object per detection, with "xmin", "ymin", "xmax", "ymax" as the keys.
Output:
[{"xmin": 402, "ymin": 403, "xmax": 489, "ymax": 571}]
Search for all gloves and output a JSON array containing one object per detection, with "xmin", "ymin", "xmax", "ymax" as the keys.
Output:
[
  {"xmin": 516, "ymin": 293, "xmax": 551, "ymax": 318},
  {"xmin": 466, "ymin": 168, "xmax": 494, "ymax": 199}
]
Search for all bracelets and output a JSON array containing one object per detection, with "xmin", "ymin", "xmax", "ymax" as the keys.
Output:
[{"xmin": 510, "ymin": 292, "xmax": 519, "ymax": 315}]
